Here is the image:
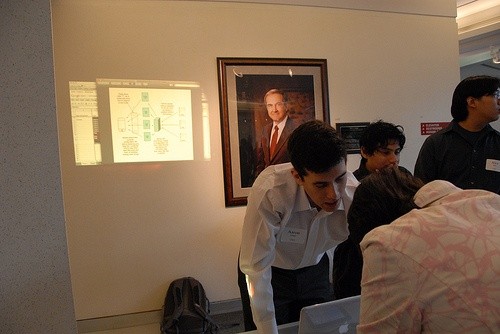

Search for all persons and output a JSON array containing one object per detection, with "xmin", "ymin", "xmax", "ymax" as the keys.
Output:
[
  {"xmin": 256, "ymin": 89, "xmax": 300, "ymax": 175},
  {"xmin": 353, "ymin": 122, "xmax": 406, "ymax": 180},
  {"xmin": 414, "ymin": 76, "xmax": 500, "ymax": 196},
  {"xmin": 347, "ymin": 166, "xmax": 500, "ymax": 334},
  {"xmin": 238, "ymin": 120, "xmax": 360, "ymax": 334}
]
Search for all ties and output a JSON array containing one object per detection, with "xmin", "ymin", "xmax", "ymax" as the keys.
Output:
[{"xmin": 270, "ymin": 125, "xmax": 279, "ymax": 161}]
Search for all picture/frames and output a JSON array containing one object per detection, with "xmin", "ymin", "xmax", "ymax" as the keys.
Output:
[
  {"xmin": 335, "ymin": 122, "xmax": 370, "ymax": 154},
  {"xmin": 216, "ymin": 57, "xmax": 331, "ymax": 209}
]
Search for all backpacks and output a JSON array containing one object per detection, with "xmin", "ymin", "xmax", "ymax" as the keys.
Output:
[{"xmin": 160, "ymin": 277, "xmax": 210, "ymax": 334}]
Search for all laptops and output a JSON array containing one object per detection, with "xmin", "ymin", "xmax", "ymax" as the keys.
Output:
[{"xmin": 298, "ymin": 295, "xmax": 360, "ymax": 334}]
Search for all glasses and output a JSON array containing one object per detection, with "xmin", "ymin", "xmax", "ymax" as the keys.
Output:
[{"xmin": 482, "ymin": 91, "xmax": 500, "ymax": 100}]
